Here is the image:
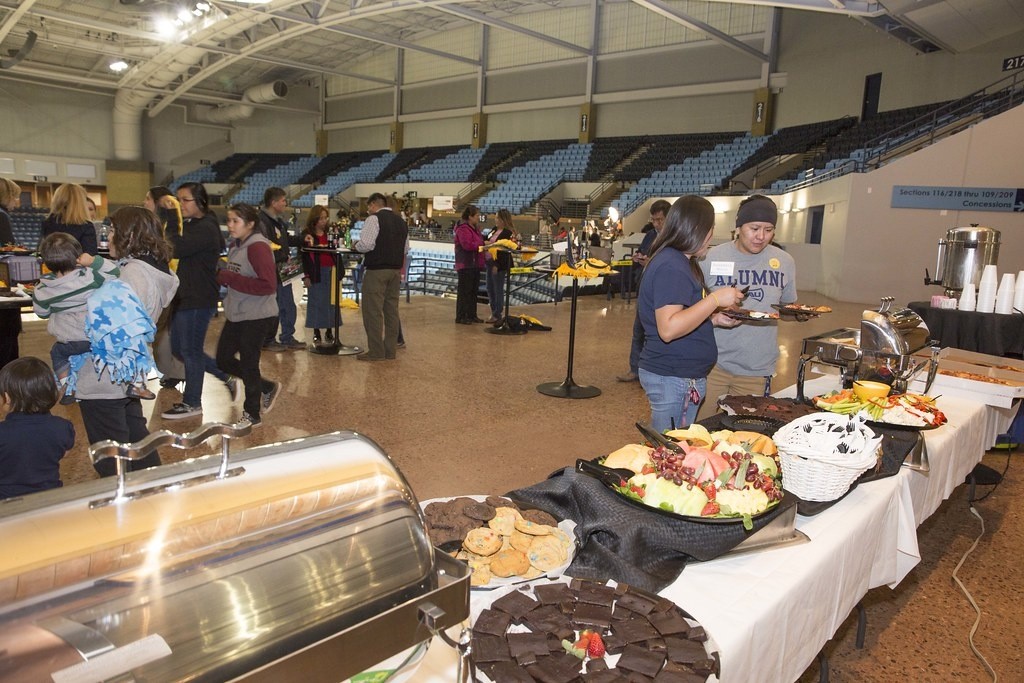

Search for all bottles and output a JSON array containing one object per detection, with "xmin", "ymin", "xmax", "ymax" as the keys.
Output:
[
  {"xmin": 100, "ymin": 226, "xmax": 107, "ymax": 248},
  {"xmin": 327, "ymin": 221, "xmax": 350, "ymax": 249}
]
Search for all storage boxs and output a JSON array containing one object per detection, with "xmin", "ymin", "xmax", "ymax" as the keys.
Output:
[{"xmin": 915, "ymin": 346, "xmax": 1024, "ymax": 397}]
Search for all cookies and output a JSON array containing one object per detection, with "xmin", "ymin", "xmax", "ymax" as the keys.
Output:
[{"xmin": 422, "ymin": 495, "xmax": 573, "ymax": 584}]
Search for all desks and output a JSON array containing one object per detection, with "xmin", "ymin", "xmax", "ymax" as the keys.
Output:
[
  {"xmin": 303, "ymin": 246, "xmax": 365, "ymax": 357},
  {"xmin": 622, "ymin": 244, "xmax": 640, "ymax": 299},
  {"xmin": 906, "ymin": 301, "xmax": 1024, "ymax": 356},
  {"xmin": 339, "ymin": 374, "xmax": 1021, "ymax": 683},
  {"xmin": 479, "ymin": 245, "xmax": 539, "ymax": 334},
  {"xmin": 532, "ymin": 265, "xmax": 621, "ymax": 398}
]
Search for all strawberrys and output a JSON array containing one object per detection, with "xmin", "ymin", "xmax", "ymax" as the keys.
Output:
[
  {"xmin": 642, "ymin": 463, "xmax": 654, "ymax": 474},
  {"xmin": 587, "ymin": 632, "xmax": 605, "ymax": 659},
  {"xmin": 703, "ymin": 480, "xmax": 716, "ymax": 501},
  {"xmin": 630, "ymin": 486, "xmax": 645, "ymax": 497},
  {"xmin": 571, "ymin": 637, "xmax": 589, "ymax": 654},
  {"xmin": 701, "ymin": 499, "xmax": 720, "ymax": 516},
  {"xmin": 580, "ymin": 629, "xmax": 593, "ymax": 644}
]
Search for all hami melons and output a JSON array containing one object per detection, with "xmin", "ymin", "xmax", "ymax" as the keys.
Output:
[{"xmin": 728, "ymin": 431, "xmax": 778, "ymax": 455}]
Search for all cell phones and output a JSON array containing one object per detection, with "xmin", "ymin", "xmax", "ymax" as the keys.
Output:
[{"xmin": 638, "ymin": 258, "xmax": 645, "ymax": 260}]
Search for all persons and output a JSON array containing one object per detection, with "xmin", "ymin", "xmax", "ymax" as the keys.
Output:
[
  {"xmin": 483, "ymin": 209, "xmax": 516, "ymax": 323},
  {"xmin": 160, "ymin": 181, "xmax": 241, "ymax": 420},
  {"xmin": 31, "ymin": 183, "xmax": 186, "ymax": 478},
  {"xmin": 299, "ymin": 205, "xmax": 344, "ymax": 343},
  {"xmin": 417, "ymin": 211, "xmax": 455, "ymax": 239},
  {"xmin": 0, "ymin": 176, "xmax": 22, "ymax": 367},
  {"xmin": 351, "ymin": 193, "xmax": 411, "ymax": 360},
  {"xmin": 540, "ymin": 217, "xmax": 622, "ymax": 248},
  {"xmin": 215, "ymin": 187, "xmax": 306, "ymax": 426},
  {"xmin": 453, "ymin": 205, "xmax": 485, "ymax": 324},
  {"xmin": 617, "ymin": 200, "xmax": 673, "ymax": 382},
  {"xmin": 695, "ymin": 194, "xmax": 820, "ymax": 422},
  {"xmin": 0, "ymin": 355, "xmax": 76, "ymax": 498},
  {"xmin": 337, "ymin": 205, "xmax": 358, "ymax": 229},
  {"xmin": 636, "ymin": 195, "xmax": 744, "ymax": 434}
]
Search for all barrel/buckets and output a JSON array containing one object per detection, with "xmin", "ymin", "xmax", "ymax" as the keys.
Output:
[{"xmin": 941, "ymin": 222, "xmax": 1002, "ymax": 291}]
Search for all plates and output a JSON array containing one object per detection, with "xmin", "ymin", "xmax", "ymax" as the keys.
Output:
[
  {"xmin": 720, "ymin": 309, "xmax": 781, "ymax": 320},
  {"xmin": 1, "ymin": 250, "xmax": 38, "ymax": 256},
  {"xmin": 597, "ymin": 478, "xmax": 783, "ymax": 524},
  {"xmin": 418, "ymin": 495, "xmax": 579, "ymax": 588},
  {"xmin": 719, "ymin": 413, "xmax": 788, "ymax": 439},
  {"xmin": 770, "ymin": 303, "xmax": 832, "ymax": 314}
]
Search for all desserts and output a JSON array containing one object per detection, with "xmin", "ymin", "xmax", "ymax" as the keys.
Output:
[
  {"xmin": 719, "ymin": 394, "xmax": 821, "ymax": 422},
  {"xmin": 468, "ymin": 579, "xmax": 715, "ymax": 683}
]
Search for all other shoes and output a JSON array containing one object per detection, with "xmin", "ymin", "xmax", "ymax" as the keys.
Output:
[
  {"xmin": 616, "ymin": 371, "xmax": 639, "ymax": 382},
  {"xmin": 470, "ymin": 315, "xmax": 484, "ymax": 322},
  {"xmin": 486, "ymin": 314, "xmax": 499, "ymax": 323},
  {"xmin": 397, "ymin": 341, "xmax": 405, "ymax": 348},
  {"xmin": 313, "ymin": 335, "xmax": 321, "ymax": 342},
  {"xmin": 455, "ymin": 318, "xmax": 472, "ymax": 324},
  {"xmin": 159, "ymin": 375, "xmax": 183, "ymax": 389},
  {"xmin": 325, "ymin": 332, "xmax": 333, "ymax": 342}
]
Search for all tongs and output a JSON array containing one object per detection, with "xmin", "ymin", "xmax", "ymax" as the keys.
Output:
[
  {"xmin": 635, "ymin": 421, "xmax": 687, "ymax": 456},
  {"xmin": 574, "ymin": 458, "xmax": 635, "ymax": 488},
  {"xmin": 731, "ymin": 282, "xmax": 750, "ymax": 293}
]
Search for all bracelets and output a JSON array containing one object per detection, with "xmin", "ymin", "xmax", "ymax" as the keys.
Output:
[
  {"xmin": 795, "ymin": 314, "xmax": 809, "ymax": 322},
  {"xmin": 710, "ymin": 292, "xmax": 719, "ymax": 306}
]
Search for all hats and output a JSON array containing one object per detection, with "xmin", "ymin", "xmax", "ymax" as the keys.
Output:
[{"xmin": 736, "ymin": 193, "xmax": 778, "ymax": 228}]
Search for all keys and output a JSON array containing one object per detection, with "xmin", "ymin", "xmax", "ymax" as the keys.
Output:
[{"xmin": 688, "ymin": 379, "xmax": 700, "ymax": 404}]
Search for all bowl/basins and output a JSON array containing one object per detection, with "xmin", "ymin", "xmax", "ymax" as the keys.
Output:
[
  {"xmin": 665, "ymin": 430, "xmax": 714, "ymax": 451},
  {"xmin": 853, "ymin": 381, "xmax": 891, "ymax": 403}
]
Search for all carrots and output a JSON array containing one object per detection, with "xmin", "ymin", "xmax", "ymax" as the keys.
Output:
[{"xmin": 812, "ymin": 389, "xmax": 855, "ymax": 403}]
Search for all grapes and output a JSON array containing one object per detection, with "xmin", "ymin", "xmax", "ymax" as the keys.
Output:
[
  {"xmin": 650, "ymin": 445, "xmax": 698, "ymax": 489},
  {"xmin": 721, "ymin": 450, "xmax": 783, "ymax": 502}
]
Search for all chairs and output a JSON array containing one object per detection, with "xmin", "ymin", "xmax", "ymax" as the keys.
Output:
[
  {"xmin": 168, "ymin": 89, "xmax": 1024, "ymax": 220},
  {"xmin": 10, "ymin": 205, "xmax": 50, "ymax": 251},
  {"xmin": 302, "ymin": 221, "xmax": 623, "ymax": 305}
]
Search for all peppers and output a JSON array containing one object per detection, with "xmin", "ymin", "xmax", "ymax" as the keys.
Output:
[{"xmin": 868, "ymin": 394, "xmax": 945, "ymax": 426}]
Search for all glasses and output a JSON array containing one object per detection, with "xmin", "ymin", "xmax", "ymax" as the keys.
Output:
[
  {"xmin": 648, "ymin": 216, "xmax": 666, "ymax": 223},
  {"xmin": 177, "ymin": 198, "xmax": 195, "ymax": 205}
]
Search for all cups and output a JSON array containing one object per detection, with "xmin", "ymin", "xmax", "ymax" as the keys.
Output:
[{"xmin": 958, "ymin": 265, "xmax": 1024, "ymax": 315}]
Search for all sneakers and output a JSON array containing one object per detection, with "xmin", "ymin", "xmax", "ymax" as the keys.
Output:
[
  {"xmin": 385, "ymin": 353, "xmax": 396, "ymax": 360},
  {"xmin": 126, "ymin": 383, "xmax": 156, "ymax": 399},
  {"xmin": 236, "ymin": 411, "xmax": 261, "ymax": 429},
  {"xmin": 260, "ymin": 381, "xmax": 282, "ymax": 414},
  {"xmin": 60, "ymin": 388, "xmax": 76, "ymax": 405},
  {"xmin": 262, "ymin": 341, "xmax": 286, "ymax": 352},
  {"xmin": 356, "ymin": 351, "xmax": 386, "ymax": 361},
  {"xmin": 283, "ymin": 337, "xmax": 307, "ymax": 349},
  {"xmin": 223, "ymin": 373, "xmax": 237, "ymax": 402},
  {"xmin": 160, "ymin": 402, "xmax": 202, "ymax": 419}
]
